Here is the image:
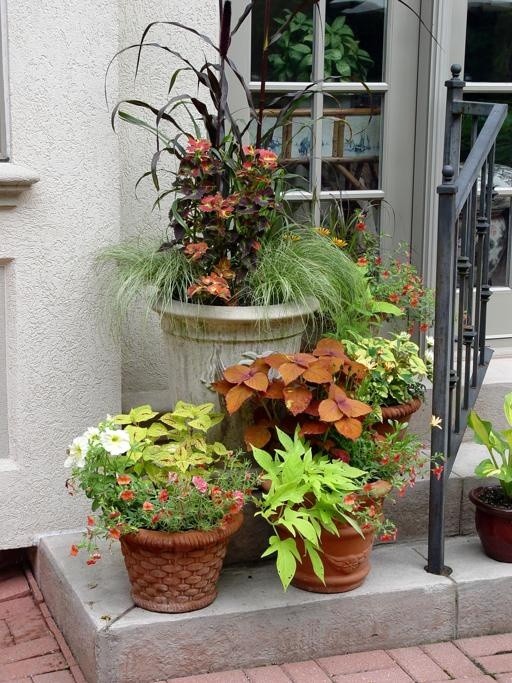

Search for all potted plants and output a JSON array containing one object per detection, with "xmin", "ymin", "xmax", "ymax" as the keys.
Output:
[{"xmin": 63, "ymin": 0, "xmax": 448, "ymax": 614}]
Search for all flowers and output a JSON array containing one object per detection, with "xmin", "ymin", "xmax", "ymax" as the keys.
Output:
[{"xmin": 469, "ymin": 391, "xmax": 512, "ymax": 566}]
